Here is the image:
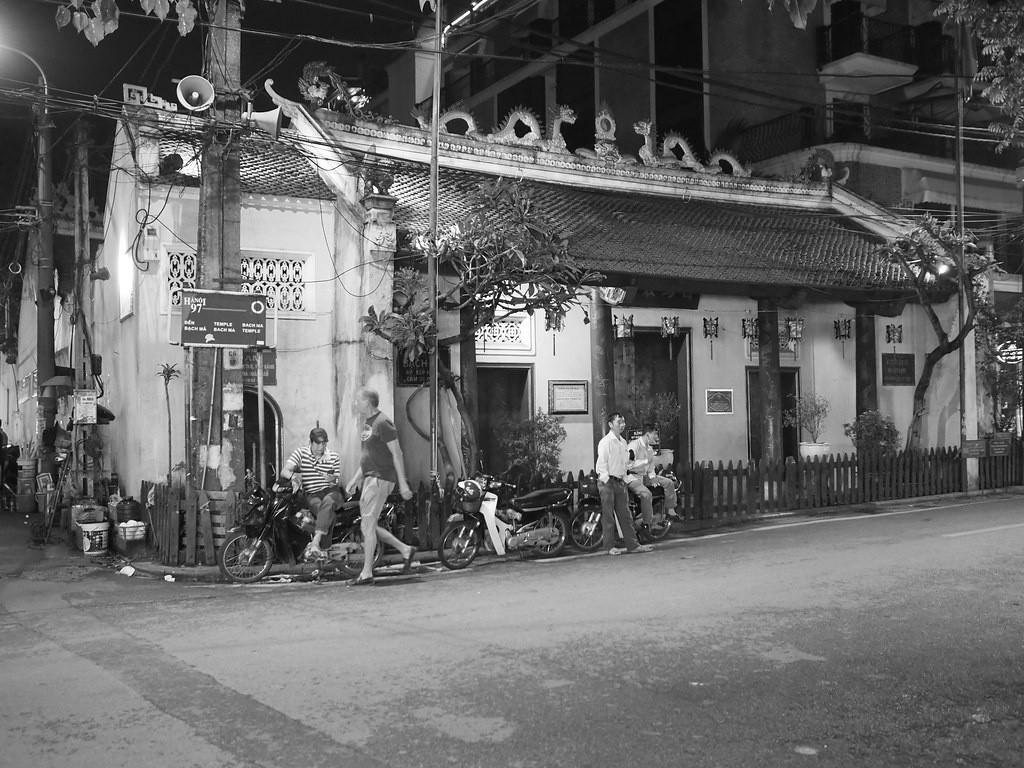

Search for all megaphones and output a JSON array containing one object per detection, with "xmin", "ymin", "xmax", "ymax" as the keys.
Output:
[{"xmin": 177, "ymin": 75, "xmax": 215, "ymax": 112}]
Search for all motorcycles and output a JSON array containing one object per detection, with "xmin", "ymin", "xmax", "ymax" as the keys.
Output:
[
  {"xmin": 217, "ymin": 461, "xmax": 400, "ymax": 584},
  {"xmin": 437, "ymin": 449, "xmax": 573, "ymax": 572},
  {"xmin": 569, "ymin": 469, "xmax": 683, "ymax": 554}
]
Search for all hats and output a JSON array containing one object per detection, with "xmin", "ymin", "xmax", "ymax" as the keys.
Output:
[{"xmin": 311, "ymin": 428, "xmax": 329, "ymax": 442}]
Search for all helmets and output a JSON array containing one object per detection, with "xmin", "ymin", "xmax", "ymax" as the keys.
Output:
[{"xmin": 455, "ymin": 479, "xmax": 481, "ymax": 502}]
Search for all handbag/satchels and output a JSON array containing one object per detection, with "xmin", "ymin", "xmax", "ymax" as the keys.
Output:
[{"xmin": 242, "ymin": 495, "xmax": 269, "ymax": 524}]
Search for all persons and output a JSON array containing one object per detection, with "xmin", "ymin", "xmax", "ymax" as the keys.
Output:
[
  {"xmin": 596, "ymin": 413, "xmax": 653, "ymax": 555},
  {"xmin": 281, "ymin": 428, "xmax": 344, "ymax": 561},
  {"xmin": 0, "ymin": 420, "xmax": 8, "ymax": 471},
  {"xmin": 345, "ymin": 388, "xmax": 417, "ymax": 585},
  {"xmin": 627, "ymin": 425, "xmax": 687, "ymax": 540}
]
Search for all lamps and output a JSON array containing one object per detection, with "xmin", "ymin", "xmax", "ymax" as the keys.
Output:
[{"xmin": 90, "ymin": 267, "xmax": 110, "ymax": 280}]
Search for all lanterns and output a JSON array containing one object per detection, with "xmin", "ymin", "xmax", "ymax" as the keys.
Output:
[
  {"xmin": 886, "ymin": 325, "xmax": 902, "ymax": 360},
  {"xmin": 546, "ymin": 309, "xmax": 561, "ymax": 356},
  {"xmin": 785, "ymin": 317, "xmax": 804, "ymax": 361},
  {"xmin": 742, "ymin": 319, "xmax": 759, "ymax": 361},
  {"xmin": 834, "ymin": 319, "xmax": 850, "ymax": 359},
  {"xmin": 475, "ymin": 307, "xmax": 493, "ymax": 352},
  {"xmin": 614, "ymin": 313, "xmax": 634, "ymax": 363},
  {"xmin": 661, "ymin": 316, "xmax": 680, "ymax": 359},
  {"xmin": 703, "ymin": 317, "xmax": 718, "ymax": 359}
]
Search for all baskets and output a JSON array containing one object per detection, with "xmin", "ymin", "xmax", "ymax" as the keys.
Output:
[
  {"xmin": 453, "ymin": 489, "xmax": 482, "ymax": 515},
  {"xmin": 236, "ymin": 500, "xmax": 270, "ymax": 525},
  {"xmin": 582, "ymin": 474, "xmax": 599, "ymax": 496}
]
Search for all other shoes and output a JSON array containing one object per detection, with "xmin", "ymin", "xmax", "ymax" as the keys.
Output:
[
  {"xmin": 666, "ymin": 514, "xmax": 687, "ymax": 523},
  {"xmin": 608, "ymin": 548, "xmax": 621, "ymax": 555},
  {"xmin": 403, "ymin": 546, "xmax": 417, "ymax": 570},
  {"xmin": 641, "ymin": 522, "xmax": 654, "ymax": 540},
  {"xmin": 346, "ymin": 576, "xmax": 374, "ymax": 586},
  {"xmin": 630, "ymin": 546, "xmax": 654, "ymax": 553},
  {"xmin": 318, "ymin": 551, "xmax": 328, "ymax": 560},
  {"xmin": 304, "ymin": 543, "xmax": 322, "ymax": 560}
]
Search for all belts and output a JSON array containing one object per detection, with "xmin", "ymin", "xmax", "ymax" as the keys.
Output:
[{"xmin": 609, "ymin": 475, "xmax": 622, "ymax": 482}]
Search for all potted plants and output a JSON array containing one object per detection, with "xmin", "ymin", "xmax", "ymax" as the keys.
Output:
[
  {"xmin": 783, "ymin": 393, "xmax": 831, "ymax": 465},
  {"xmin": 630, "ymin": 390, "xmax": 684, "ymax": 470}
]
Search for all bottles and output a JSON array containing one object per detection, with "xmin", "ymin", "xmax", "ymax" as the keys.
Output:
[{"xmin": 118, "ymin": 496, "xmax": 142, "ymax": 524}]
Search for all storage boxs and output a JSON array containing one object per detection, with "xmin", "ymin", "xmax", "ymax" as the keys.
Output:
[{"xmin": 14, "ymin": 459, "xmax": 36, "ymax": 511}]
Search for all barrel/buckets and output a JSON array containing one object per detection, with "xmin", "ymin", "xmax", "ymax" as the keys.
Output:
[{"xmin": 81, "ymin": 522, "xmax": 109, "ymax": 554}]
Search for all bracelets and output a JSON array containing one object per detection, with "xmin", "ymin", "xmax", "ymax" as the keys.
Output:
[{"xmin": 289, "ymin": 473, "xmax": 294, "ymax": 479}]
